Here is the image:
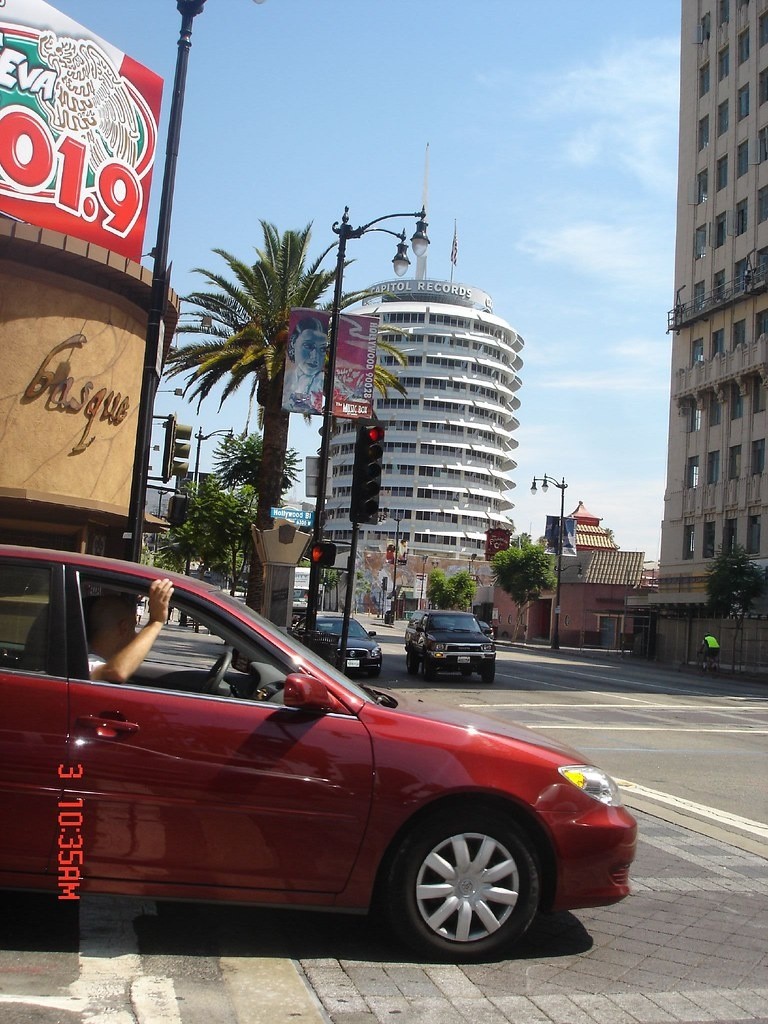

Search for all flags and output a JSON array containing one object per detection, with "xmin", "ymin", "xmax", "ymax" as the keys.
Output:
[{"xmin": 451, "ymin": 219, "xmax": 458, "ymax": 266}]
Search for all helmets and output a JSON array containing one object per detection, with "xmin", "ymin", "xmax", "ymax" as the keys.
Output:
[{"xmin": 705, "ymin": 633, "xmax": 711, "ymax": 636}]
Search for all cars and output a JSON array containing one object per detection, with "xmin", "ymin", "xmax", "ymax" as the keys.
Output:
[
  {"xmin": 478, "ymin": 620, "xmax": 494, "ymax": 641},
  {"xmin": 292, "ymin": 615, "xmax": 383, "ymax": 677},
  {"xmin": 0, "ymin": 544, "xmax": 638, "ymax": 962}
]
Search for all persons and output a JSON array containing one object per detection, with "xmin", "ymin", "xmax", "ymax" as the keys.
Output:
[
  {"xmin": 88, "ymin": 579, "xmax": 175, "ymax": 682},
  {"xmin": 282, "ymin": 316, "xmax": 328, "ymax": 413},
  {"xmin": 698, "ymin": 633, "xmax": 720, "ymax": 673},
  {"xmin": 137, "ymin": 594, "xmax": 173, "ymax": 625}
]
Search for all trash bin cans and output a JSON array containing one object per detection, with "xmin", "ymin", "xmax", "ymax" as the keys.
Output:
[
  {"xmin": 404, "ymin": 611, "xmax": 409, "ymax": 619},
  {"xmin": 490, "ymin": 625, "xmax": 498, "ymax": 639},
  {"xmin": 385, "ymin": 611, "xmax": 394, "ymax": 624},
  {"xmin": 407, "ymin": 611, "xmax": 414, "ymax": 619}
]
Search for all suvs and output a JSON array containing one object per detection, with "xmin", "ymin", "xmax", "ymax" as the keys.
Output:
[{"xmin": 405, "ymin": 610, "xmax": 496, "ymax": 683}]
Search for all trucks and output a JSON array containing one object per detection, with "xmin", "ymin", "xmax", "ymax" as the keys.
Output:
[{"xmin": 292, "ymin": 567, "xmax": 311, "ymax": 614}]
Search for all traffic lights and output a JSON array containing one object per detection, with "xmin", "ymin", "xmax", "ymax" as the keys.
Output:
[
  {"xmin": 349, "ymin": 417, "xmax": 385, "ymax": 525},
  {"xmin": 162, "ymin": 413, "xmax": 192, "ymax": 483},
  {"xmin": 381, "ymin": 577, "xmax": 387, "ymax": 590},
  {"xmin": 310, "ymin": 542, "xmax": 336, "ymax": 566}
]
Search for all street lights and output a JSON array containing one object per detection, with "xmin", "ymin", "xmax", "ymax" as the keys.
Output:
[
  {"xmin": 308, "ymin": 206, "xmax": 431, "ymax": 646},
  {"xmin": 179, "ymin": 426, "xmax": 234, "ymax": 626},
  {"xmin": 530, "ymin": 473, "xmax": 568, "ymax": 649}
]
